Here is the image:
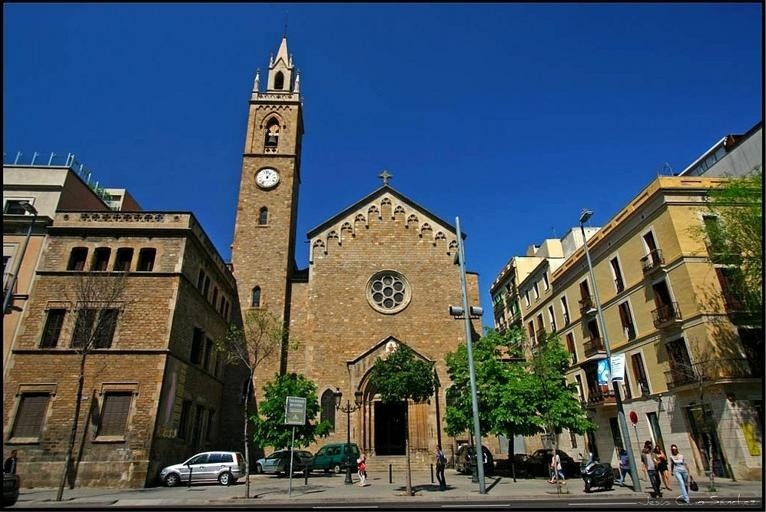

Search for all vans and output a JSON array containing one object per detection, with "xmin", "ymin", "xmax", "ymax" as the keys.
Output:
[
  {"xmin": 314, "ymin": 444, "xmax": 361, "ymax": 475},
  {"xmin": 159, "ymin": 451, "xmax": 246, "ymax": 486}
]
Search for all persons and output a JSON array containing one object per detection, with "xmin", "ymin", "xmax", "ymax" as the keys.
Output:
[
  {"xmin": 4, "ymin": 449, "xmax": 18, "ymax": 474},
  {"xmin": 619, "ymin": 448, "xmax": 633, "ymax": 487},
  {"xmin": 357, "ymin": 454, "xmax": 368, "ymax": 487},
  {"xmin": 434, "ymin": 445, "xmax": 447, "ymax": 491},
  {"xmin": 546, "ymin": 451, "xmax": 565, "ymax": 483},
  {"xmin": 640, "ymin": 441, "xmax": 661, "ymax": 499},
  {"xmin": 669, "ymin": 445, "xmax": 691, "ymax": 503},
  {"xmin": 653, "ymin": 445, "xmax": 672, "ymax": 492}
]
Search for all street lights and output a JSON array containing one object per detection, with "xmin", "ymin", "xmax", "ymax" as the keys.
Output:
[
  {"xmin": 579, "ymin": 207, "xmax": 642, "ymax": 491},
  {"xmin": 333, "ymin": 390, "xmax": 363, "ymax": 485},
  {"xmin": 2, "ymin": 201, "xmax": 38, "ymax": 313}
]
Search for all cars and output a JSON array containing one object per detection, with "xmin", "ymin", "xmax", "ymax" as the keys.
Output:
[
  {"xmin": 525, "ymin": 449, "xmax": 574, "ymax": 475},
  {"xmin": 254, "ymin": 450, "xmax": 315, "ymax": 477},
  {"xmin": 456, "ymin": 445, "xmax": 495, "ymax": 474}
]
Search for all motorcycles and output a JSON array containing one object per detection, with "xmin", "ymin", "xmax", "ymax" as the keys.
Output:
[{"xmin": 579, "ymin": 452, "xmax": 614, "ymax": 492}]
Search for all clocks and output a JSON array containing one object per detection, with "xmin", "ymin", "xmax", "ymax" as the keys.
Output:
[{"xmin": 254, "ymin": 168, "xmax": 280, "ymax": 190}]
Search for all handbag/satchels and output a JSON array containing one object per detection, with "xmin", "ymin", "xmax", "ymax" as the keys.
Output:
[{"xmin": 690, "ymin": 476, "xmax": 698, "ymax": 490}]
